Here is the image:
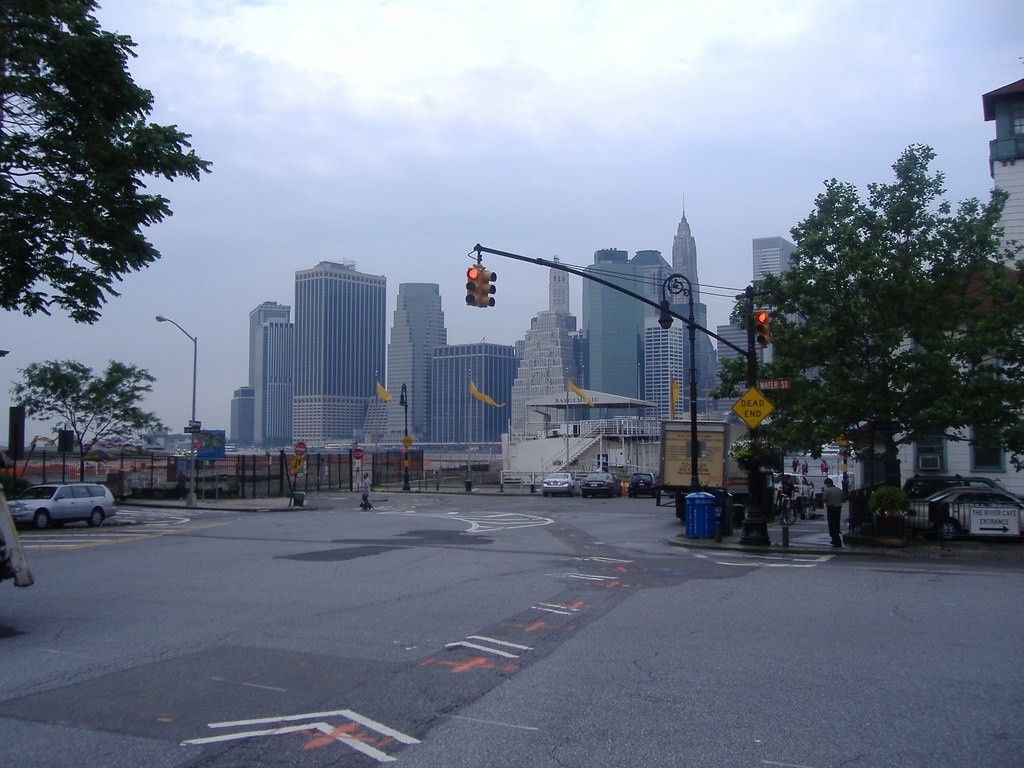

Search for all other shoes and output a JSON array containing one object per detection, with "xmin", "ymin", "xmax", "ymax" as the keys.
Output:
[
  {"xmin": 832, "ymin": 545, "xmax": 842, "ymax": 547},
  {"xmin": 830, "ymin": 541, "xmax": 834, "ymax": 544}
]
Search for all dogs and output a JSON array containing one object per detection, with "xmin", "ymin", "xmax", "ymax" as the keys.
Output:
[{"xmin": 360, "ymin": 502, "xmax": 374, "ymax": 512}]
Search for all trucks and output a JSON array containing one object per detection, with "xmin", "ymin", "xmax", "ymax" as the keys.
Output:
[{"xmin": 656, "ymin": 419, "xmax": 775, "ymax": 527}]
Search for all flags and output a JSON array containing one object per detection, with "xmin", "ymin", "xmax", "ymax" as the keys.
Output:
[
  {"xmin": 376, "ymin": 382, "xmax": 393, "ymax": 401},
  {"xmin": 671, "ymin": 379, "xmax": 679, "ymax": 420},
  {"xmin": 568, "ymin": 380, "xmax": 594, "ymax": 407},
  {"xmin": 471, "ymin": 382, "xmax": 504, "ymax": 407}
]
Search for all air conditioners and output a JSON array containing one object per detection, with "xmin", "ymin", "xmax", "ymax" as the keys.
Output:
[{"xmin": 919, "ymin": 456, "xmax": 941, "ymax": 469}]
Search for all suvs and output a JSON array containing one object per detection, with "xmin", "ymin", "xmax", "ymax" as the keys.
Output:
[{"xmin": 7, "ymin": 482, "xmax": 117, "ymax": 529}]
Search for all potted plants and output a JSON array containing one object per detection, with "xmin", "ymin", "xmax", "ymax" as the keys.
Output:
[{"xmin": 868, "ymin": 486, "xmax": 910, "ymax": 539}]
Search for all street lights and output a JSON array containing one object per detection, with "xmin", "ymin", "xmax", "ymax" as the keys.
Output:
[
  {"xmin": 156, "ymin": 316, "xmax": 197, "ymax": 507},
  {"xmin": 399, "ymin": 383, "xmax": 410, "ymax": 490},
  {"xmin": 658, "ymin": 274, "xmax": 700, "ymax": 493}
]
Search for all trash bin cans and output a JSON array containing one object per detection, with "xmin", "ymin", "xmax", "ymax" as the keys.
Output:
[
  {"xmin": 684, "ymin": 492, "xmax": 717, "ymax": 538},
  {"xmin": 709, "ymin": 491, "xmax": 733, "ymax": 536},
  {"xmin": 465, "ymin": 480, "xmax": 472, "ymax": 492},
  {"xmin": 293, "ymin": 492, "xmax": 305, "ymax": 507}
]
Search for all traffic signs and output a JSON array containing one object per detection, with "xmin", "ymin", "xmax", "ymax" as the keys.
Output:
[
  {"xmin": 184, "ymin": 420, "xmax": 201, "ymax": 434},
  {"xmin": 969, "ymin": 506, "xmax": 1020, "ymax": 537}
]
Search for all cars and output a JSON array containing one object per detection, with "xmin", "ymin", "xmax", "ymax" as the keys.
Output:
[
  {"xmin": 581, "ymin": 473, "xmax": 621, "ymax": 497},
  {"xmin": 542, "ymin": 473, "xmax": 586, "ymax": 497},
  {"xmin": 628, "ymin": 473, "xmax": 657, "ymax": 498},
  {"xmin": 773, "ymin": 473, "xmax": 814, "ymax": 502},
  {"xmin": 902, "ymin": 473, "xmax": 1024, "ymax": 542}
]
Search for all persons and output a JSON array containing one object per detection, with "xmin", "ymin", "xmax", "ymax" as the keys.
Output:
[
  {"xmin": 793, "ymin": 457, "xmax": 808, "ymax": 475},
  {"xmin": 821, "ymin": 459, "xmax": 828, "ymax": 475},
  {"xmin": 822, "ymin": 478, "xmax": 843, "ymax": 546},
  {"xmin": 362, "ymin": 473, "xmax": 373, "ymax": 511},
  {"xmin": 782, "ymin": 475, "xmax": 795, "ymax": 499}
]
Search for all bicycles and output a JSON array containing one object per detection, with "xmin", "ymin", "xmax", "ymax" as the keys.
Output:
[{"xmin": 782, "ymin": 498, "xmax": 813, "ymax": 525}]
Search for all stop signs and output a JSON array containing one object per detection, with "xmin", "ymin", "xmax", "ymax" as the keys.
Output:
[
  {"xmin": 295, "ymin": 442, "xmax": 307, "ymax": 456},
  {"xmin": 353, "ymin": 448, "xmax": 364, "ymax": 459}
]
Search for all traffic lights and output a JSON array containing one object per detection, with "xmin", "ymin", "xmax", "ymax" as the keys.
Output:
[
  {"xmin": 480, "ymin": 270, "xmax": 497, "ymax": 308},
  {"xmin": 754, "ymin": 312, "xmax": 770, "ymax": 346},
  {"xmin": 465, "ymin": 268, "xmax": 480, "ymax": 308}
]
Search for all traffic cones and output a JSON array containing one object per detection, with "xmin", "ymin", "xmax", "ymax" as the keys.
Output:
[{"xmin": 622, "ymin": 480, "xmax": 626, "ymax": 494}]
